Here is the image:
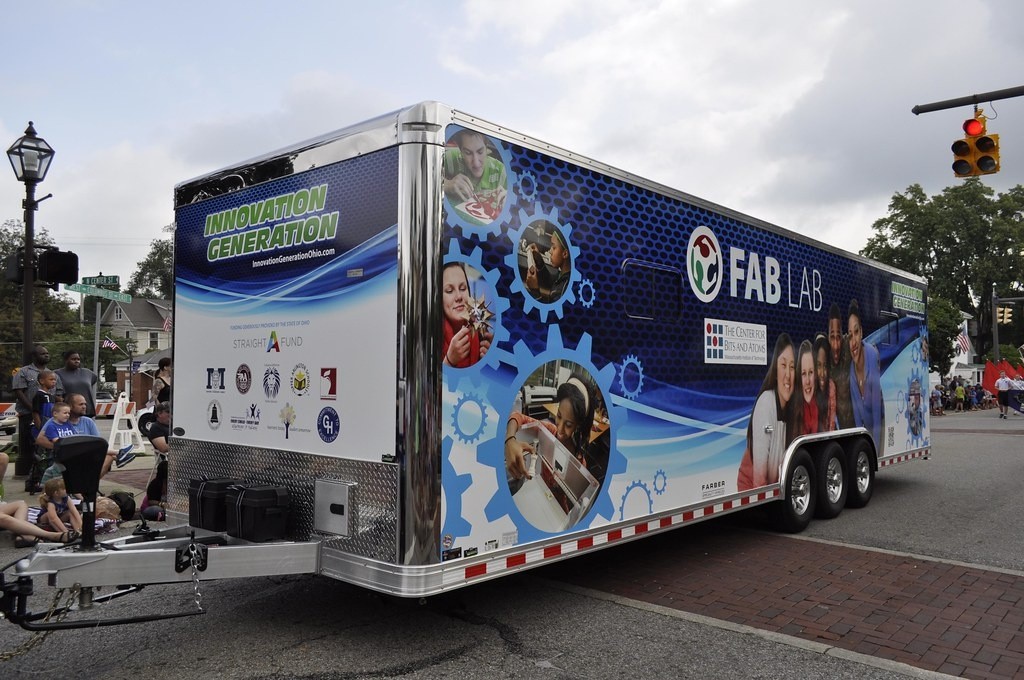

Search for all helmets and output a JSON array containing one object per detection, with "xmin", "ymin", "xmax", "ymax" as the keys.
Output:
[{"xmin": 908, "ymin": 379, "xmax": 921, "ymax": 405}]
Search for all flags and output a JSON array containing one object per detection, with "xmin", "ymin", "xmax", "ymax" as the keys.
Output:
[
  {"xmin": 102, "ymin": 338, "xmax": 117, "ymax": 349},
  {"xmin": 982, "ymin": 360, "xmax": 1024, "ymax": 413},
  {"xmin": 957, "ymin": 327, "xmax": 970, "ymax": 354},
  {"xmin": 164, "ymin": 317, "xmax": 173, "ymax": 331}
]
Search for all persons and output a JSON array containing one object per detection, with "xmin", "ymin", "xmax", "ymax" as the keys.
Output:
[
  {"xmin": 738, "ymin": 299, "xmax": 885, "ymax": 492},
  {"xmin": 0, "ymin": 452, "xmax": 83, "ymax": 548},
  {"xmin": 442, "ymin": 129, "xmax": 507, "ymax": 202},
  {"xmin": 1013, "ymin": 374, "xmax": 1024, "ymax": 416},
  {"xmin": 13, "ymin": 345, "xmax": 135, "ymax": 494},
  {"xmin": 140, "ymin": 461, "xmax": 168, "ymax": 522},
  {"xmin": 525, "ymin": 229, "xmax": 570, "ymax": 303},
  {"xmin": 910, "ymin": 380, "xmax": 923, "ymax": 435},
  {"xmin": 151, "ymin": 358, "xmax": 171, "ymax": 406},
  {"xmin": 150, "ymin": 401, "xmax": 171, "ymax": 451},
  {"xmin": 995, "ymin": 370, "xmax": 1012, "ymax": 419},
  {"xmin": 504, "ymin": 373, "xmax": 596, "ymax": 514},
  {"xmin": 930, "ymin": 375, "xmax": 999, "ymax": 416},
  {"xmin": 439, "ymin": 262, "xmax": 494, "ymax": 368}
]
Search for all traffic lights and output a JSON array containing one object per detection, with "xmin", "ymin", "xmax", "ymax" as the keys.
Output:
[
  {"xmin": 1004, "ymin": 308, "xmax": 1012, "ymax": 323},
  {"xmin": 997, "ymin": 306, "xmax": 1003, "ymax": 322},
  {"xmin": 951, "ymin": 116, "xmax": 1000, "ymax": 177}
]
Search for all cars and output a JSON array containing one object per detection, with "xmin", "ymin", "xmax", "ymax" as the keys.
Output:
[{"xmin": 97, "ymin": 391, "xmax": 117, "ymax": 403}]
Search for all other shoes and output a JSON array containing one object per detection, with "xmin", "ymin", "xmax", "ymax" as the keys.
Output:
[
  {"xmin": 1004, "ymin": 416, "xmax": 1007, "ymax": 419},
  {"xmin": 25, "ymin": 484, "xmax": 42, "ymax": 492},
  {"xmin": 1017, "ymin": 412, "xmax": 1020, "ymax": 416},
  {"xmin": 1014, "ymin": 411, "xmax": 1017, "ymax": 415},
  {"xmin": 999, "ymin": 414, "xmax": 1004, "ymax": 419},
  {"xmin": 931, "ymin": 403, "xmax": 998, "ymax": 416}
]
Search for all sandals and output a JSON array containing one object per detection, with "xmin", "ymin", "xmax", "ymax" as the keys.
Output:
[
  {"xmin": 15, "ymin": 534, "xmax": 46, "ymax": 548},
  {"xmin": 58, "ymin": 531, "xmax": 79, "ymax": 543}
]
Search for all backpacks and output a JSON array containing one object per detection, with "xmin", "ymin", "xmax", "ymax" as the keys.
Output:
[{"xmin": 931, "ymin": 390, "xmax": 939, "ymax": 401}]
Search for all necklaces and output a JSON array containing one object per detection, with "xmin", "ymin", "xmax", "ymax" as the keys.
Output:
[{"xmin": 855, "ymin": 365, "xmax": 863, "ymax": 386}]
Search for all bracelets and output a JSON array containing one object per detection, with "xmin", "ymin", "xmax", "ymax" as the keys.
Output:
[
  {"xmin": 446, "ymin": 354, "xmax": 453, "ymax": 367},
  {"xmin": 505, "ymin": 435, "xmax": 516, "ymax": 443}
]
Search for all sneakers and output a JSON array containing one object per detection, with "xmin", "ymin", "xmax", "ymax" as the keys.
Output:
[
  {"xmin": 116, "ymin": 444, "xmax": 135, "ymax": 461},
  {"xmin": 116, "ymin": 454, "xmax": 135, "ymax": 468}
]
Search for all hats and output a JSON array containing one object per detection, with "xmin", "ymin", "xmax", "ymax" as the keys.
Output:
[{"xmin": 156, "ymin": 401, "xmax": 169, "ymax": 412}]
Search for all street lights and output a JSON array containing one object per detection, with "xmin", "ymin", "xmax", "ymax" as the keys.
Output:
[
  {"xmin": 5, "ymin": 121, "xmax": 55, "ymax": 481},
  {"xmin": 126, "ymin": 338, "xmax": 135, "ymax": 402}
]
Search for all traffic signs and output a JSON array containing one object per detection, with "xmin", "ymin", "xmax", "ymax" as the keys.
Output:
[{"xmin": 63, "ymin": 275, "xmax": 132, "ymax": 305}]
